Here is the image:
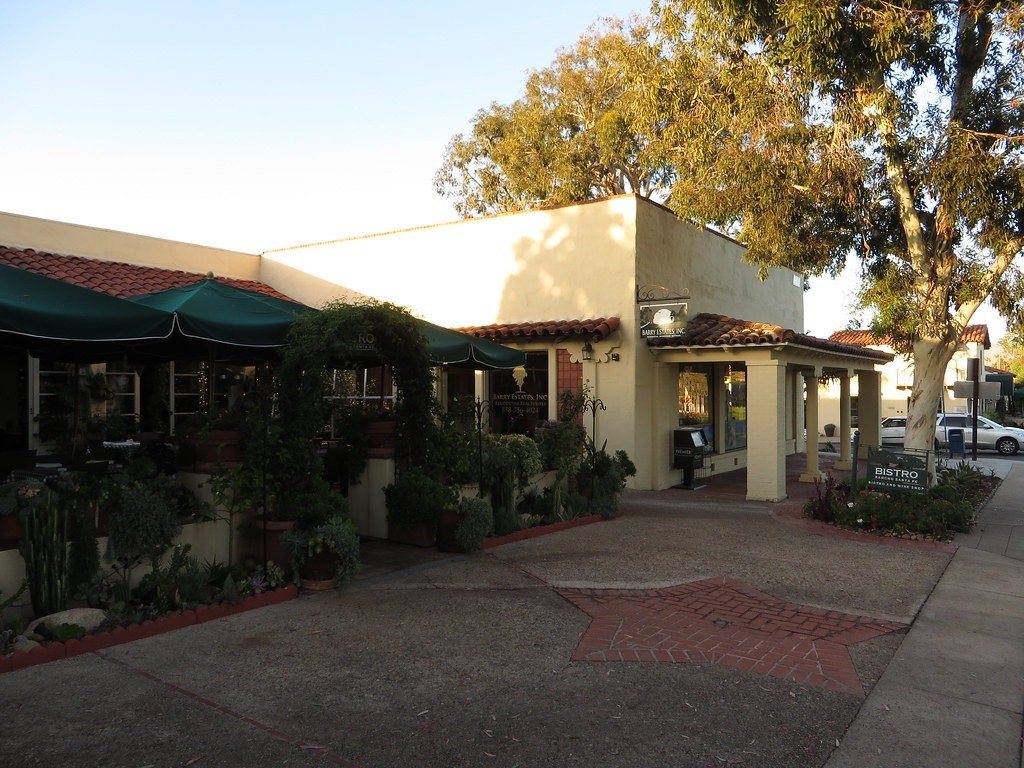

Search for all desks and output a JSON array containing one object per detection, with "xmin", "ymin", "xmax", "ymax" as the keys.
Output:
[
  {"xmin": 103, "ymin": 442, "xmax": 141, "ymax": 465},
  {"xmin": 11, "ymin": 467, "xmax": 67, "ymax": 484}
]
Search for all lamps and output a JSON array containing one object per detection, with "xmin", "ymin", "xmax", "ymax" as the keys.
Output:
[{"xmin": 582, "ymin": 336, "xmax": 592, "ymax": 360}]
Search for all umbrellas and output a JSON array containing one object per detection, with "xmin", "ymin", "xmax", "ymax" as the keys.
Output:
[{"xmin": 0, "ymin": 264, "xmax": 527, "ymax": 472}]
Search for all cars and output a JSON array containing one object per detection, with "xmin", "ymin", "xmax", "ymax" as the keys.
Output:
[
  {"xmin": 932, "ymin": 413, "xmax": 1024, "ymax": 455},
  {"xmin": 850, "ymin": 413, "xmax": 941, "ymax": 444}
]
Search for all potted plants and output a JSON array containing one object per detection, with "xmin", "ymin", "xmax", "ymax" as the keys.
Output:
[
  {"xmin": 0, "ymin": 361, "xmax": 635, "ymax": 642},
  {"xmin": 824, "ymin": 424, "xmax": 836, "ymax": 436}
]
